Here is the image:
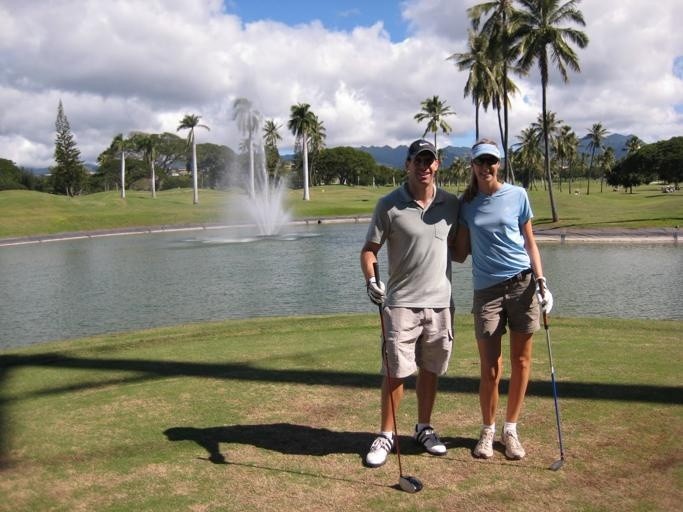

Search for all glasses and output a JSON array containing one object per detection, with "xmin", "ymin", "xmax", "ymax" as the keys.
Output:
[{"xmin": 473, "ymin": 157, "xmax": 498, "ymax": 165}]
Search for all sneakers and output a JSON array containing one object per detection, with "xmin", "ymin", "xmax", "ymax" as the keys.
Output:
[
  {"xmin": 366, "ymin": 434, "xmax": 395, "ymax": 468},
  {"xmin": 500, "ymin": 426, "xmax": 525, "ymax": 460},
  {"xmin": 413, "ymin": 424, "xmax": 447, "ymax": 455},
  {"xmin": 472, "ymin": 427, "xmax": 495, "ymax": 458}
]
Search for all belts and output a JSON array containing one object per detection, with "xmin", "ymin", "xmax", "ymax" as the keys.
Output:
[{"xmin": 512, "ymin": 268, "xmax": 532, "ymax": 281}]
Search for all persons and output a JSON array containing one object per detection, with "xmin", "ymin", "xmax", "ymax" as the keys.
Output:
[
  {"xmin": 450, "ymin": 139, "xmax": 553, "ymax": 460},
  {"xmin": 362, "ymin": 139, "xmax": 461, "ymax": 467}
]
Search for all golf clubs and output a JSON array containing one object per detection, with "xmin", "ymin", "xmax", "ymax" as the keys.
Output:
[
  {"xmin": 540, "ymin": 282, "xmax": 565, "ymax": 471},
  {"xmin": 373, "ymin": 262, "xmax": 423, "ymax": 493}
]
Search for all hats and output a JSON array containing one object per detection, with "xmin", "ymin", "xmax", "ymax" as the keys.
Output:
[
  {"xmin": 470, "ymin": 143, "xmax": 500, "ymax": 162},
  {"xmin": 409, "ymin": 139, "xmax": 438, "ymax": 162}
]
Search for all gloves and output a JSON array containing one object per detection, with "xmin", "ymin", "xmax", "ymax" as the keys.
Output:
[
  {"xmin": 535, "ymin": 276, "xmax": 554, "ymax": 314},
  {"xmin": 367, "ymin": 277, "xmax": 385, "ymax": 305}
]
